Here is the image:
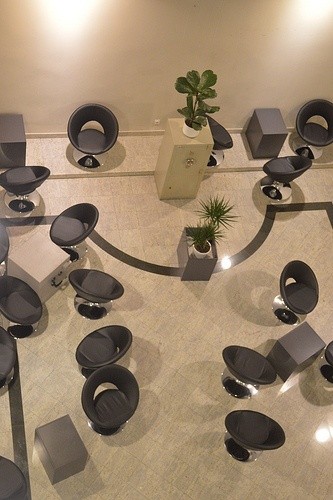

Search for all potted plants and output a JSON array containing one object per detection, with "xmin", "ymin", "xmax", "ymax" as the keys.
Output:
[
  {"xmin": 175, "ymin": 67, "xmax": 220, "ymax": 137},
  {"xmin": 182, "ymin": 193, "xmax": 241, "ymax": 259}
]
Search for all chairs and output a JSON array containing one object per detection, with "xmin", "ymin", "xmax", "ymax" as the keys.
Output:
[{"xmin": 0, "ymin": 99, "xmax": 333, "ymax": 500}]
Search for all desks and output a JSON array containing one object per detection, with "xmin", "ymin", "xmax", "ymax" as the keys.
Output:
[
  {"xmin": 0, "ymin": 114, "xmax": 26, "ymax": 169},
  {"xmin": 8, "ymin": 235, "xmax": 71, "ymax": 304},
  {"xmin": 244, "ymin": 108, "xmax": 287, "ymax": 159},
  {"xmin": 33, "ymin": 415, "xmax": 89, "ymax": 486},
  {"xmin": 153, "ymin": 117, "xmax": 214, "ymax": 200},
  {"xmin": 176, "ymin": 228, "xmax": 218, "ymax": 282},
  {"xmin": 265, "ymin": 321, "xmax": 327, "ymax": 384}
]
